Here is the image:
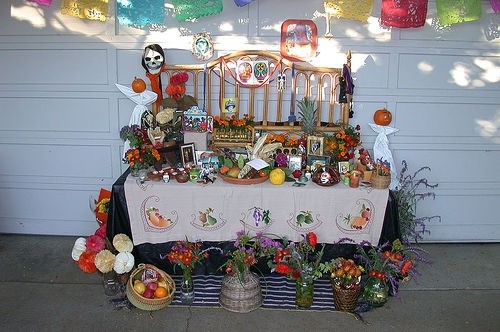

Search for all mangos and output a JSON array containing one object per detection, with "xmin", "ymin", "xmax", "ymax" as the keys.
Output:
[{"xmin": 227, "ymin": 167, "xmax": 240, "ymax": 177}]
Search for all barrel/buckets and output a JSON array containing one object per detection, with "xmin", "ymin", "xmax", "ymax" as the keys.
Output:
[{"xmin": 370, "ymin": 173, "xmax": 391, "ymax": 189}]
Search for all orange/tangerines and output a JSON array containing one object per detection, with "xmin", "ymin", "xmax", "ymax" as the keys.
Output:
[{"xmin": 155, "ymin": 287, "xmax": 167, "ymax": 297}]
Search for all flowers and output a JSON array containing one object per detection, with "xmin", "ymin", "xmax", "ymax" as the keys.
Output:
[{"xmin": 72, "ymin": 72, "xmax": 442, "ymax": 296}]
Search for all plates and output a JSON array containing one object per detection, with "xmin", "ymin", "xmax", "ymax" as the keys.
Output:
[{"xmin": 217, "ymin": 172, "xmax": 268, "ymax": 185}]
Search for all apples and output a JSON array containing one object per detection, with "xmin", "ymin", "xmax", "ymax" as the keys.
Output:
[{"xmin": 144, "ymin": 282, "xmax": 157, "ymax": 298}]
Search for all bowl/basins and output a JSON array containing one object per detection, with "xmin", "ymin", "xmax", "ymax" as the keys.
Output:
[{"xmin": 147, "ymin": 168, "xmax": 190, "ymax": 183}]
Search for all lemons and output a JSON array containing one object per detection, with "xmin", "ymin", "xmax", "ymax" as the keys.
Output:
[{"xmin": 134, "ymin": 282, "xmax": 145, "ymax": 294}]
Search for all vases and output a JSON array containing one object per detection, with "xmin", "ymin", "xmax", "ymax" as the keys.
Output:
[
  {"xmin": 296, "ymin": 280, "xmax": 313, "ymax": 308},
  {"xmin": 332, "ymin": 285, "xmax": 361, "ymax": 312},
  {"xmin": 131, "ymin": 164, "xmax": 138, "ymax": 177},
  {"xmin": 180, "ymin": 274, "xmax": 195, "ymax": 305},
  {"xmin": 363, "ymin": 276, "xmax": 389, "ymax": 307}
]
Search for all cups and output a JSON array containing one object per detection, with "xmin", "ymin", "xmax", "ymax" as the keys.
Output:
[
  {"xmin": 138, "ymin": 170, "xmax": 147, "ymax": 181},
  {"xmin": 350, "ymin": 176, "xmax": 359, "ymax": 188}
]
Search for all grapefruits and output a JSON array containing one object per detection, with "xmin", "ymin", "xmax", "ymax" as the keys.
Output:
[{"xmin": 270, "ymin": 168, "xmax": 285, "ymax": 185}]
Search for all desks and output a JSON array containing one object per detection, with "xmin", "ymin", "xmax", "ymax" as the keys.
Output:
[{"xmin": 105, "ymin": 164, "xmax": 401, "ymax": 275}]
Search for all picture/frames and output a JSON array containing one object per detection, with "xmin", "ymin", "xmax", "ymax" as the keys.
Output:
[
  {"xmin": 307, "ymin": 136, "xmax": 324, "ymax": 156},
  {"xmin": 192, "ymin": 32, "xmax": 214, "ymax": 60},
  {"xmin": 306, "ymin": 155, "xmax": 331, "ymax": 173},
  {"xmin": 280, "ymin": 19, "xmax": 318, "ymax": 62},
  {"xmin": 179, "ymin": 143, "xmax": 197, "ymax": 167},
  {"xmin": 338, "ymin": 161, "xmax": 350, "ymax": 175}
]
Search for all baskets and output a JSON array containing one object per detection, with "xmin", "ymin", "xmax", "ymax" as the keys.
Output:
[
  {"xmin": 218, "ymin": 272, "xmax": 263, "ymax": 314},
  {"xmin": 332, "ymin": 286, "xmax": 357, "ymax": 312},
  {"xmin": 218, "ymin": 171, "xmax": 269, "ymax": 185},
  {"xmin": 371, "ymin": 158, "xmax": 392, "ymax": 189},
  {"xmin": 356, "ymin": 161, "xmax": 372, "ymax": 180},
  {"xmin": 124, "ymin": 263, "xmax": 176, "ymax": 311}
]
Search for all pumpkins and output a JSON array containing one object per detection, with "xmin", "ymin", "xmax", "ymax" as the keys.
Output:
[{"xmin": 132, "ymin": 76, "xmax": 146, "ymax": 93}]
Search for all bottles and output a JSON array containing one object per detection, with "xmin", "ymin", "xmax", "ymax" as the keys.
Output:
[
  {"xmin": 162, "ymin": 172, "xmax": 169, "ymax": 183},
  {"xmin": 383, "ymin": 160, "xmax": 389, "ymax": 169},
  {"xmin": 190, "ymin": 168, "xmax": 199, "ymax": 183},
  {"xmin": 305, "ymin": 170, "xmax": 311, "ymax": 181}
]
